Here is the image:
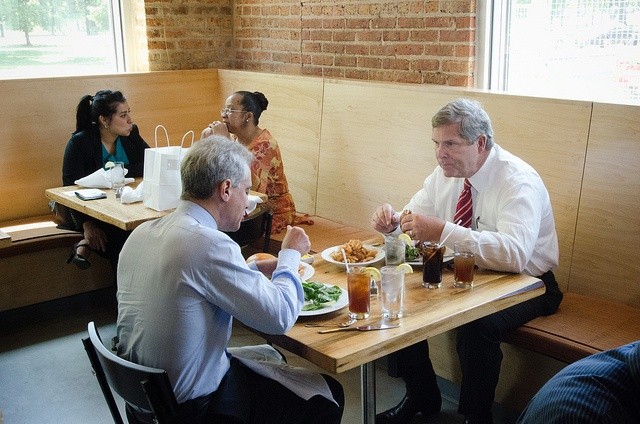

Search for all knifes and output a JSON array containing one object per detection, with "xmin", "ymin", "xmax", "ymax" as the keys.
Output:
[{"xmin": 317, "ymin": 320, "xmax": 400, "ymax": 334}]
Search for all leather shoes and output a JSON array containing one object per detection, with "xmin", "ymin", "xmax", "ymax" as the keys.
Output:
[
  {"xmin": 376, "ymin": 386, "xmax": 442, "ymax": 424},
  {"xmin": 463, "ymin": 412, "xmax": 492, "ymax": 424}
]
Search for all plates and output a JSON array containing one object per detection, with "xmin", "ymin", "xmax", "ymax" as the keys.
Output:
[
  {"xmin": 297, "ymin": 259, "xmax": 315, "ymax": 281},
  {"xmin": 296, "ymin": 280, "xmax": 353, "ymax": 316},
  {"xmin": 321, "ymin": 241, "xmax": 385, "ymax": 270},
  {"xmin": 383, "ymin": 239, "xmax": 444, "ymax": 265}
]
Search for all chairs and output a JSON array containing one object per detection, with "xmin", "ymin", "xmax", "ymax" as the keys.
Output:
[{"xmin": 77, "ymin": 322, "xmax": 184, "ymax": 424}]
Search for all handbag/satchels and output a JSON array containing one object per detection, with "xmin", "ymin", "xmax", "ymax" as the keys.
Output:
[{"xmin": 141, "ymin": 125, "xmax": 195, "ymax": 211}]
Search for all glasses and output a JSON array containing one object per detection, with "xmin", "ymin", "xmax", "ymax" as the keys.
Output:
[{"xmin": 221, "ymin": 107, "xmax": 247, "ymax": 115}]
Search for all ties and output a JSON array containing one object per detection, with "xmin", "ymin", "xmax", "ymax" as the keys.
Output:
[{"xmin": 452, "ymin": 179, "xmax": 473, "ymax": 228}]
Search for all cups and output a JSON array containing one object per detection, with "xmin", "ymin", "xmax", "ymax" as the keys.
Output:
[
  {"xmin": 384, "ymin": 236, "xmax": 406, "ymax": 266},
  {"xmin": 346, "ymin": 265, "xmax": 372, "ymax": 320},
  {"xmin": 110, "ymin": 161, "xmax": 125, "ymax": 199},
  {"xmin": 452, "ymin": 238, "xmax": 477, "ymax": 290},
  {"xmin": 420, "ymin": 241, "xmax": 444, "ymax": 289},
  {"xmin": 380, "ymin": 265, "xmax": 406, "ymax": 320}
]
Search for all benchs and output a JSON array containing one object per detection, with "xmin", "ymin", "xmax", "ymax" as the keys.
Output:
[
  {"xmin": 0, "ymin": 69, "xmax": 218, "ymax": 333},
  {"xmin": 217, "ymin": 65, "xmax": 640, "ymax": 422}
]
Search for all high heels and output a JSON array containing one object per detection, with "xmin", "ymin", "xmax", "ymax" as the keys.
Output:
[{"xmin": 64, "ymin": 239, "xmax": 92, "ymax": 269}]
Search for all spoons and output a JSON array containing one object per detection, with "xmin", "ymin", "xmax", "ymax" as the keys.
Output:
[{"xmin": 303, "ymin": 317, "xmax": 357, "ymax": 327}]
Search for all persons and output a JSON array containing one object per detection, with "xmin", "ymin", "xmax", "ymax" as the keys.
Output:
[
  {"xmin": 370, "ymin": 98, "xmax": 565, "ymax": 424},
  {"xmin": 201, "ymin": 89, "xmax": 314, "ymax": 248},
  {"xmin": 115, "ymin": 134, "xmax": 346, "ymax": 422},
  {"xmin": 515, "ymin": 338, "xmax": 638, "ymax": 423},
  {"xmin": 55, "ymin": 89, "xmax": 152, "ymax": 275}
]
